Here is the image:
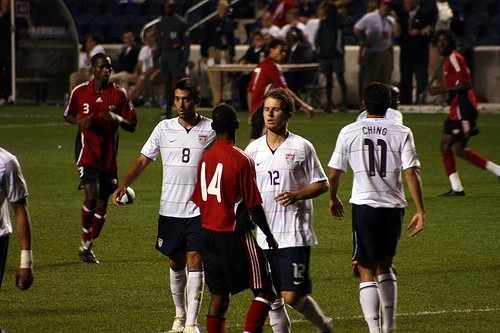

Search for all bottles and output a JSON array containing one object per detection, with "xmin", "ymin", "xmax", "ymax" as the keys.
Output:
[
  {"xmin": 259, "ymin": 52, "xmax": 265, "ymax": 62},
  {"xmin": 219, "ymin": 50, "xmax": 227, "ymax": 65}
]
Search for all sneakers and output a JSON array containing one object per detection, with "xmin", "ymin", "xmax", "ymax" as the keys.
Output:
[{"xmin": 78, "ymin": 249, "xmax": 99, "ymax": 264}]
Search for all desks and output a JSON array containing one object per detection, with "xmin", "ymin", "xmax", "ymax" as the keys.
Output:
[{"xmin": 208, "ymin": 63, "xmax": 322, "ymax": 115}]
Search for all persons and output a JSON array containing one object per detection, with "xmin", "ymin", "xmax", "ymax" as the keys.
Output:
[
  {"xmin": 0, "ymin": 0, "xmax": 17, "ymax": 105},
  {"xmin": 248, "ymin": 39, "xmax": 313, "ymax": 145},
  {"xmin": 0, "ymin": 147, "xmax": 34, "ymax": 290},
  {"xmin": 112, "ymin": 77, "xmax": 216, "ymax": 333},
  {"xmin": 66, "ymin": 0, "xmax": 479, "ymax": 118},
  {"xmin": 327, "ymin": 82, "xmax": 425, "ymax": 333},
  {"xmin": 245, "ymin": 87, "xmax": 332, "ymax": 333},
  {"xmin": 430, "ymin": 29, "xmax": 500, "ymax": 196},
  {"xmin": 196, "ymin": 103, "xmax": 279, "ymax": 333},
  {"xmin": 63, "ymin": 53, "xmax": 137, "ymax": 263}
]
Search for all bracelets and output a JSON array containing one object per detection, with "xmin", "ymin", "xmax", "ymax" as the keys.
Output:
[{"xmin": 19, "ymin": 249, "xmax": 32, "ymax": 269}]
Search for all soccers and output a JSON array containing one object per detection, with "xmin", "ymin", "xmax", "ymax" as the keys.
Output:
[{"xmin": 116, "ymin": 185, "xmax": 135, "ymax": 205}]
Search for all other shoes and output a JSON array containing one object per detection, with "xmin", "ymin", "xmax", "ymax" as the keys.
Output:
[
  {"xmin": 172, "ymin": 316, "xmax": 185, "ymax": 333},
  {"xmin": 326, "ymin": 105, "xmax": 332, "ymax": 113},
  {"xmin": 338, "ymin": 106, "xmax": 348, "ymax": 112},
  {"xmin": 438, "ymin": 189, "xmax": 465, "ymax": 197},
  {"xmin": 184, "ymin": 323, "xmax": 201, "ymax": 333}
]
